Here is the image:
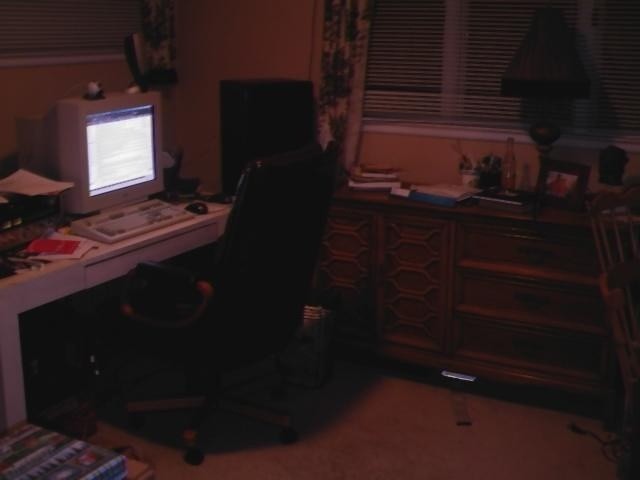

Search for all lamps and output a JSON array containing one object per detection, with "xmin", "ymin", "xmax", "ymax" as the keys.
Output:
[{"xmin": 499, "ymin": 8, "xmax": 591, "ymax": 207}]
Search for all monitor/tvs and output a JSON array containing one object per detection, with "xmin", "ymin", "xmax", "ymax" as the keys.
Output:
[{"xmin": 16, "ymin": 92, "xmax": 165, "ymax": 215}]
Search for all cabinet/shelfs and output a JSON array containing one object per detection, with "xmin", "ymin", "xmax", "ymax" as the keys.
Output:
[{"xmin": 309, "ymin": 173, "xmax": 639, "ymax": 428}]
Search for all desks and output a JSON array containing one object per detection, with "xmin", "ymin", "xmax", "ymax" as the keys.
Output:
[{"xmin": 0, "ymin": 191, "xmax": 234, "ymax": 432}]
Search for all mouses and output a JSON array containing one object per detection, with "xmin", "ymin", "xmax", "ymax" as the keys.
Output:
[{"xmin": 185, "ymin": 203, "xmax": 208, "ymax": 214}]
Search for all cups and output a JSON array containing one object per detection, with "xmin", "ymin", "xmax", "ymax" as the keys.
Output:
[{"xmin": 461, "ymin": 169, "xmax": 481, "ymax": 188}]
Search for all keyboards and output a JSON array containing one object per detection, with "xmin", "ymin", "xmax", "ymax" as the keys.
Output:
[{"xmin": 70, "ymin": 198, "xmax": 195, "ymax": 245}]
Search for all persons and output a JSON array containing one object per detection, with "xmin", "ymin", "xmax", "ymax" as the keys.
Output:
[{"xmin": 547, "ymin": 171, "xmax": 569, "ymax": 198}]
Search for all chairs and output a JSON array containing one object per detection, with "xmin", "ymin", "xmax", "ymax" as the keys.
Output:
[{"xmin": 57, "ymin": 143, "xmax": 340, "ymax": 466}]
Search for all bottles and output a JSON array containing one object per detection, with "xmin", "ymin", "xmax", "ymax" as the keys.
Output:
[{"xmin": 500, "ymin": 136, "xmax": 518, "ymax": 189}]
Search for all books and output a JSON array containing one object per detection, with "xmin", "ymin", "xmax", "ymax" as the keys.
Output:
[{"xmin": 347, "ymin": 162, "xmax": 543, "ymax": 214}]
